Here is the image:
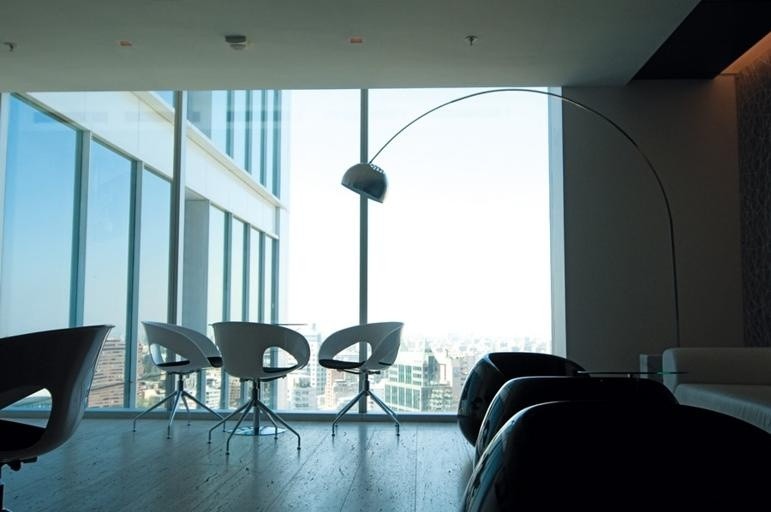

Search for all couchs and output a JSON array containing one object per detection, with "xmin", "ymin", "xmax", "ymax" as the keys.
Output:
[{"xmin": 662, "ymin": 347, "xmax": 771, "ymax": 434}]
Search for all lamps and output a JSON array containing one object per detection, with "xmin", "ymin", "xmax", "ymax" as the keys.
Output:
[{"xmin": 341, "ymin": 88, "xmax": 681, "ymax": 347}]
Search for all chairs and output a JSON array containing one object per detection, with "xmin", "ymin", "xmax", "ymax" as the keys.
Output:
[
  {"xmin": 472, "ymin": 376, "xmax": 677, "ymax": 467},
  {"xmin": 457, "ymin": 353, "xmax": 586, "ymax": 446},
  {"xmin": 132, "ymin": 321, "xmax": 222, "ymax": 439},
  {"xmin": 208, "ymin": 321, "xmax": 310, "ymax": 455},
  {"xmin": 460, "ymin": 401, "xmax": 770, "ymax": 511},
  {"xmin": 0, "ymin": 323, "xmax": 114, "ymax": 512},
  {"xmin": 318, "ymin": 321, "xmax": 403, "ymax": 437}
]
